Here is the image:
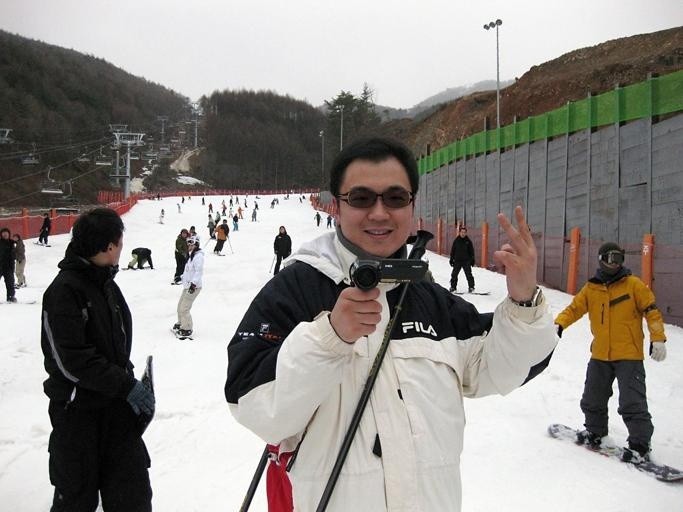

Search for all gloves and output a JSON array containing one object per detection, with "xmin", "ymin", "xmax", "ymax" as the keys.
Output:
[
  {"xmin": 648, "ymin": 341, "xmax": 665, "ymax": 361},
  {"xmin": 188, "ymin": 282, "xmax": 195, "ymax": 294},
  {"xmin": 126, "ymin": 380, "xmax": 154, "ymax": 415}
]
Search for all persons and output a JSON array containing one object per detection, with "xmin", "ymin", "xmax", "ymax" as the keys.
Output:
[
  {"xmin": 128, "ymin": 247, "xmax": 153, "ymax": 271},
  {"xmin": 10, "ymin": 233, "xmax": 26, "ymax": 288},
  {"xmin": 37, "ymin": 206, "xmax": 160, "ymax": 510},
  {"xmin": 173, "ymin": 229, "xmax": 192, "ymax": 283},
  {"xmin": 172, "ymin": 236, "xmax": 204, "ymax": 337},
  {"xmin": 221, "ymin": 135, "xmax": 558, "ymax": 511},
  {"xmin": 150, "ymin": 186, "xmax": 332, "ymax": 256},
  {"xmin": 448, "ymin": 228, "xmax": 476, "ymax": 293},
  {"xmin": 273, "ymin": 226, "xmax": 292, "ymax": 275},
  {"xmin": 551, "ymin": 242, "xmax": 668, "ymax": 463},
  {"xmin": 37, "ymin": 212, "xmax": 51, "ymax": 246},
  {"xmin": 0, "ymin": 228, "xmax": 17, "ymax": 303}
]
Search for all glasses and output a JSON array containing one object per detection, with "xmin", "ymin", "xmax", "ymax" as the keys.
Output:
[
  {"xmin": 186, "ymin": 239, "xmax": 195, "ymax": 245},
  {"xmin": 598, "ymin": 252, "xmax": 623, "ymax": 264},
  {"xmin": 335, "ymin": 187, "xmax": 413, "ymax": 208}
]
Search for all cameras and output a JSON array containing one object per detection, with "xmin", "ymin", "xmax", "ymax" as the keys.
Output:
[{"xmin": 351, "ymin": 258, "xmax": 428, "ymax": 291}]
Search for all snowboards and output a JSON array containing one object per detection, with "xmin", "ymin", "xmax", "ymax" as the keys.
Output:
[
  {"xmin": 121, "ymin": 267, "xmax": 152, "ymax": 270},
  {"xmin": 172, "ymin": 324, "xmax": 192, "ymax": 341},
  {"xmin": 449, "ymin": 289, "xmax": 494, "ymax": 296},
  {"xmin": 546, "ymin": 421, "xmax": 681, "ymax": 484},
  {"xmin": 212, "ymin": 250, "xmax": 224, "ymax": 255}
]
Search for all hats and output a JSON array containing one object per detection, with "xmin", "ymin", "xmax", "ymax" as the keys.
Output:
[{"xmin": 598, "ymin": 242, "xmax": 620, "ymax": 275}]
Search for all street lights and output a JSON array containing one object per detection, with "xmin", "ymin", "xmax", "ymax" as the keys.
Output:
[
  {"xmin": 319, "ymin": 129, "xmax": 325, "ymax": 184},
  {"xmin": 334, "ymin": 103, "xmax": 345, "ymax": 152},
  {"xmin": 483, "ymin": 18, "xmax": 502, "ymax": 128}
]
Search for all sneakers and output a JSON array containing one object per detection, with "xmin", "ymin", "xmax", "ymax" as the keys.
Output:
[
  {"xmin": 622, "ymin": 441, "xmax": 651, "ymax": 464},
  {"xmin": 176, "ymin": 329, "xmax": 191, "ymax": 336},
  {"xmin": 575, "ymin": 430, "xmax": 607, "ymax": 444}
]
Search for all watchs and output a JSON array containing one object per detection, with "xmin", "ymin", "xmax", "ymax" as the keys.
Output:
[{"xmin": 509, "ymin": 286, "xmax": 544, "ymax": 307}]
